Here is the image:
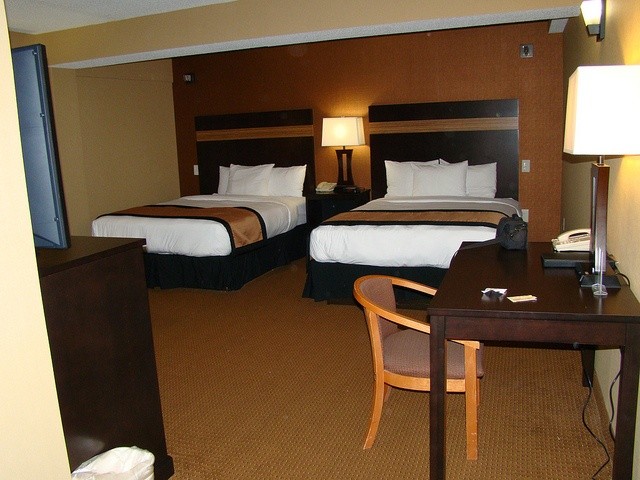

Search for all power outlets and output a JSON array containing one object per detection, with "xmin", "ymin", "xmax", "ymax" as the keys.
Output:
[{"xmin": 519, "ymin": 43, "xmax": 534, "ymax": 58}]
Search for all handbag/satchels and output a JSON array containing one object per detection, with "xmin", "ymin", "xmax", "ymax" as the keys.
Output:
[{"xmin": 495, "ymin": 214, "xmax": 528, "ymax": 250}]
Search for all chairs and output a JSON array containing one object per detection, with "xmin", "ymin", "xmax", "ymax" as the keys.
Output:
[{"xmin": 353, "ymin": 274, "xmax": 484, "ymax": 461}]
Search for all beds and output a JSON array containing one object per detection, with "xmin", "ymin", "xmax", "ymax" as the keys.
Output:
[
  {"xmin": 91, "ymin": 109, "xmax": 317, "ymax": 291},
  {"xmin": 301, "ymin": 98, "xmax": 522, "ymax": 307}
]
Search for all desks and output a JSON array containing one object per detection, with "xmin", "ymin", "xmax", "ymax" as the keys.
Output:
[{"xmin": 425, "ymin": 240, "xmax": 640, "ymax": 480}]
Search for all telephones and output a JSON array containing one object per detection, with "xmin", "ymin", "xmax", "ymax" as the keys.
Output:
[
  {"xmin": 551, "ymin": 228, "xmax": 591, "ymax": 252},
  {"xmin": 315, "ymin": 182, "xmax": 337, "ymax": 192}
]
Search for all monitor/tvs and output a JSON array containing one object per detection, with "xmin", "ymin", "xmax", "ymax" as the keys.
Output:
[{"xmin": 11, "ymin": 42, "xmax": 71, "ymax": 250}]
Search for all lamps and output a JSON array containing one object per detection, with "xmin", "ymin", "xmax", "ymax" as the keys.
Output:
[
  {"xmin": 580, "ymin": 0, "xmax": 606, "ymax": 41},
  {"xmin": 562, "ymin": 65, "xmax": 639, "ymax": 290},
  {"xmin": 321, "ymin": 117, "xmax": 366, "ymax": 194},
  {"xmin": 182, "ymin": 72, "xmax": 193, "ymax": 85}
]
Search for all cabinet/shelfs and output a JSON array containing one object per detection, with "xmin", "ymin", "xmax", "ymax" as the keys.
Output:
[{"xmin": 35, "ymin": 234, "xmax": 175, "ymax": 480}]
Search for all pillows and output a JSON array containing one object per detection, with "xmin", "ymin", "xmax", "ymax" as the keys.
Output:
[
  {"xmin": 270, "ymin": 164, "xmax": 307, "ymax": 197},
  {"xmin": 412, "ymin": 160, "xmax": 469, "ymax": 197},
  {"xmin": 439, "ymin": 158, "xmax": 497, "ymax": 199},
  {"xmin": 225, "ymin": 163, "xmax": 275, "ymax": 196},
  {"xmin": 218, "ymin": 166, "xmax": 230, "ymax": 195},
  {"xmin": 384, "ymin": 159, "xmax": 440, "ymax": 198}
]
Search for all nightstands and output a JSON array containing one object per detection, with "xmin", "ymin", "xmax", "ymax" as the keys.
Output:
[{"xmin": 307, "ymin": 186, "xmax": 371, "ymax": 230}]
